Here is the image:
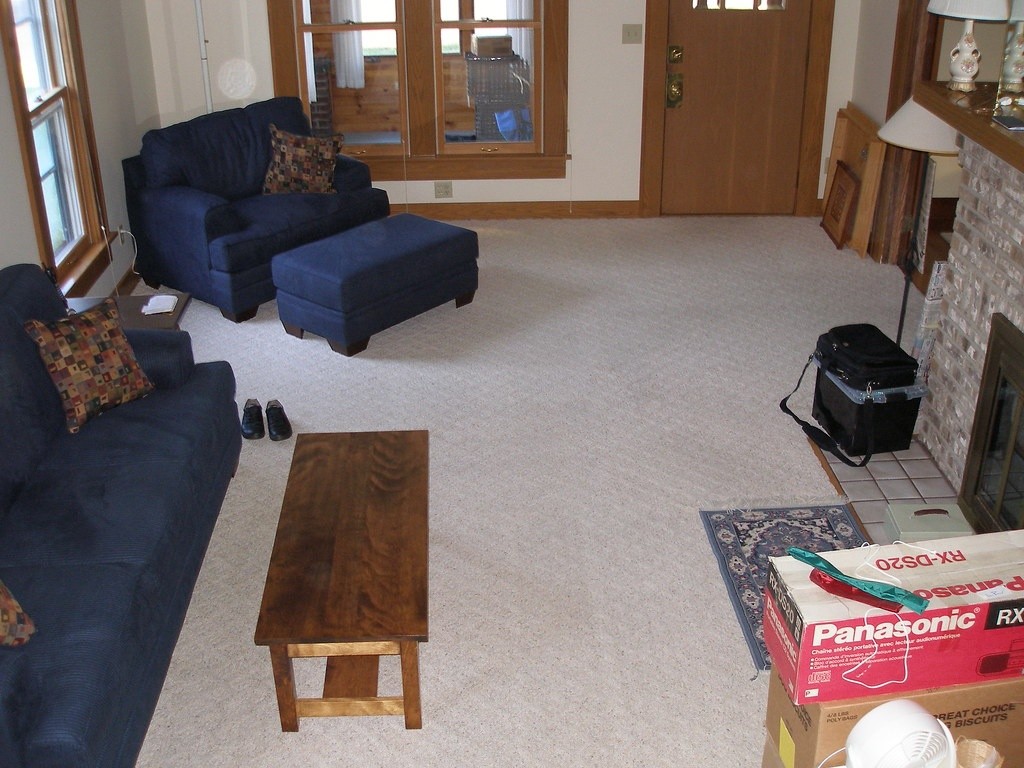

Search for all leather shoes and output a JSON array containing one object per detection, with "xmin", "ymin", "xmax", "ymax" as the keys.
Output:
[
  {"xmin": 266, "ymin": 399, "xmax": 292, "ymax": 441},
  {"xmin": 241, "ymin": 399, "xmax": 265, "ymax": 439}
]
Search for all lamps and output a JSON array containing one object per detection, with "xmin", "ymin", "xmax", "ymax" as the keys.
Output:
[
  {"xmin": 877, "ymin": 96, "xmax": 960, "ymax": 347},
  {"xmin": 927, "ymin": 0, "xmax": 1009, "ymax": 93}
]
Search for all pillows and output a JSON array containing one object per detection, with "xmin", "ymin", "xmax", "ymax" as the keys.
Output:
[
  {"xmin": 23, "ymin": 295, "xmax": 156, "ymax": 433},
  {"xmin": 0, "ymin": 579, "xmax": 35, "ymax": 648},
  {"xmin": 261, "ymin": 123, "xmax": 346, "ymax": 195}
]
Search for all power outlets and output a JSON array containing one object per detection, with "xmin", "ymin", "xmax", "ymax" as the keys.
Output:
[{"xmin": 434, "ymin": 180, "xmax": 453, "ymax": 199}]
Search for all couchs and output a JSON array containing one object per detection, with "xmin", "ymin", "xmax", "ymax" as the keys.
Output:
[
  {"xmin": 0, "ymin": 264, "xmax": 243, "ymax": 768},
  {"xmin": 121, "ymin": 98, "xmax": 391, "ymax": 323}
]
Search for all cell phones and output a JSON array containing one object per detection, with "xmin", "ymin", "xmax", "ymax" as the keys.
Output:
[{"xmin": 991, "ymin": 115, "xmax": 1024, "ymax": 131}]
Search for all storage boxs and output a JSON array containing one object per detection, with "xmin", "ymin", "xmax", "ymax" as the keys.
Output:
[
  {"xmin": 474, "ymin": 103, "xmax": 525, "ymax": 141},
  {"xmin": 463, "ymin": 51, "xmax": 530, "ymax": 104},
  {"xmin": 763, "ymin": 662, "xmax": 1024, "ymax": 768},
  {"xmin": 812, "ymin": 356, "xmax": 930, "ymax": 457},
  {"xmin": 762, "ymin": 529, "xmax": 1024, "ymax": 705},
  {"xmin": 476, "ymin": 34, "xmax": 513, "ymax": 57},
  {"xmin": 883, "ymin": 503, "xmax": 972, "ymax": 545}
]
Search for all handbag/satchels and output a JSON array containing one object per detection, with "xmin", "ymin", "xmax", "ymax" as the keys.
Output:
[{"xmin": 780, "ymin": 323, "xmax": 919, "ymax": 469}]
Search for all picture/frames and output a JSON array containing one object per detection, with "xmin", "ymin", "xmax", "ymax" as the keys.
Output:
[{"xmin": 820, "ymin": 160, "xmax": 862, "ymax": 251}]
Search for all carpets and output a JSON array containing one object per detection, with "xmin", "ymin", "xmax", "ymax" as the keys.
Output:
[{"xmin": 697, "ymin": 492, "xmax": 868, "ymax": 675}]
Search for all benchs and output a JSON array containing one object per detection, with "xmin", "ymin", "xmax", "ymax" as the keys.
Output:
[
  {"xmin": 254, "ymin": 430, "xmax": 429, "ymax": 731},
  {"xmin": 271, "ymin": 213, "xmax": 479, "ymax": 357}
]
